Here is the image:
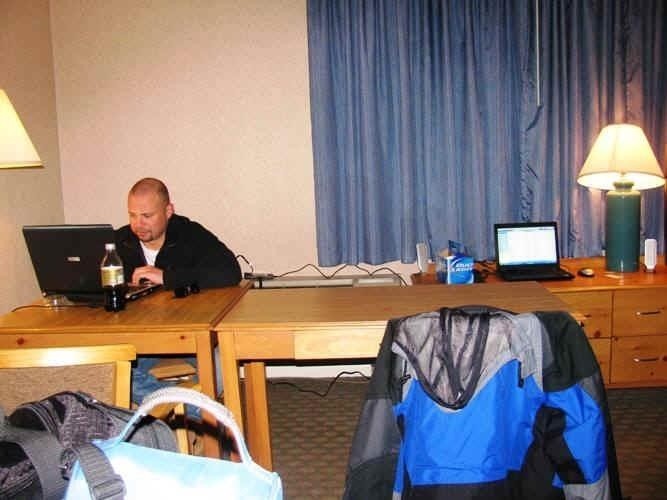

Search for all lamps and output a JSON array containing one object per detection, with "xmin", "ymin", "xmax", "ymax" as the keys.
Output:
[
  {"xmin": 575, "ymin": 122, "xmax": 666, "ymax": 275},
  {"xmin": 0, "ymin": 88, "xmax": 46, "ymax": 171}
]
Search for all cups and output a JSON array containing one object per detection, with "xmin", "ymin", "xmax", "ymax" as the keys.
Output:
[{"xmin": 46, "ymin": 294, "xmax": 67, "ymax": 314}]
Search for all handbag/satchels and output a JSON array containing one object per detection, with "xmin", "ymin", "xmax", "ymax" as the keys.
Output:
[{"xmin": 60, "ymin": 385, "xmax": 285, "ymax": 500}]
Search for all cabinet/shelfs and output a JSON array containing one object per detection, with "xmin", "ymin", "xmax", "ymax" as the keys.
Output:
[{"xmin": 405, "ymin": 256, "xmax": 666, "ymax": 392}]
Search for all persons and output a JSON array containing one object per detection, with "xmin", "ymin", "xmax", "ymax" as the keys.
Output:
[{"xmin": 113, "ymin": 176, "xmax": 243, "ymax": 463}]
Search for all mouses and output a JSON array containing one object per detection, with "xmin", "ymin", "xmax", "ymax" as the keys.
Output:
[{"xmin": 577, "ymin": 267, "xmax": 594, "ymax": 277}]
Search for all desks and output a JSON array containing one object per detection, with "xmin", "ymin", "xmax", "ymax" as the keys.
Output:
[
  {"xmin": 210, "ymin": 279, "xmax": 602, "ymax": 472},
  {"xmin": 0, "ymin": 277, "xmax": 255, "ymax": 473}
]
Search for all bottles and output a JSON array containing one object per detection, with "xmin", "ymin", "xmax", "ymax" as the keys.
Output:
[{"xmin": 101, "ymin": 244, "xmax": 125, "ymax": 312}]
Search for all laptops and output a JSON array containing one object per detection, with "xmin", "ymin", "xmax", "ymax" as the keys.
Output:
[
  {"xmin": 22, "ymin": 224, "xmax": 161, "ymax": 303},
  {"xmin": 494, "ymin": 221, "xmax": 575, "ymax": 281}
]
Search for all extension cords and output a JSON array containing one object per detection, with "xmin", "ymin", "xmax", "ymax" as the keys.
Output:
[{"xmin": 244, "ymin": 273, "xmax": 274, "ymax": 280}]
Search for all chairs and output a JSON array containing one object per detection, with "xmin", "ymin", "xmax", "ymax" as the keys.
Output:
[{"xmin": 0, "ymin": 342, "xmax": 139, "ymax": 416}]
